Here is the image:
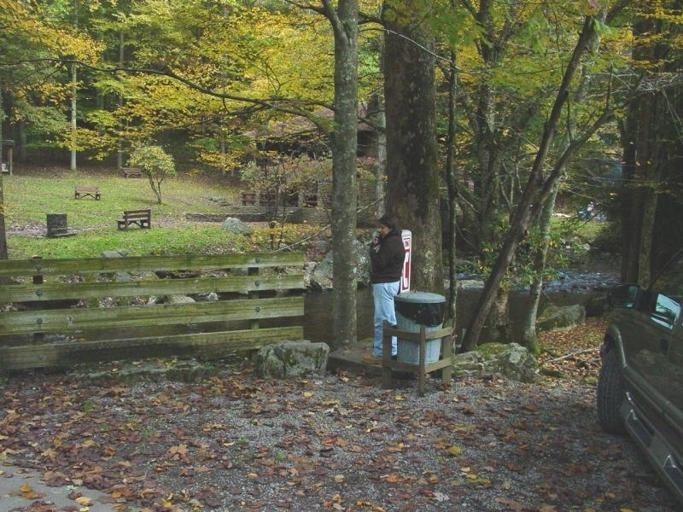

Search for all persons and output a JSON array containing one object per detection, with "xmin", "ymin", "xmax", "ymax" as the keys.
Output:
[{"xmin": 370, "ymin": 213, "xmax": 405, "ymax": 358}]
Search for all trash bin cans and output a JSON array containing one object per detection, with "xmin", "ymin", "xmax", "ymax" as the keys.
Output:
[{"xmin": 394, "ymin": 292, "xmax": 447, "ymax": 363}]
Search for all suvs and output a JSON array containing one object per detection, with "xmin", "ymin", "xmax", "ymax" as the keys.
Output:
[{"xmin": 594, "ymin": 251, "xmax": 683, "ymax": 501}]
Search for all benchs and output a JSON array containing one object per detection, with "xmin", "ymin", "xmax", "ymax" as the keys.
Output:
[
  {"xmin": 115, "ymin": 208, "xmax": 151, "ymax": 231},
  {"xmin": 75, "ymin": 185, "xmax": 101, "ymax": 201},
  {"xmin": 240, "ymin": 191, "xmax": 256, "ymax": 206},
  {"xmin": 122, "ymin": 167, "xmax": 143, "ymax": 179}
]
